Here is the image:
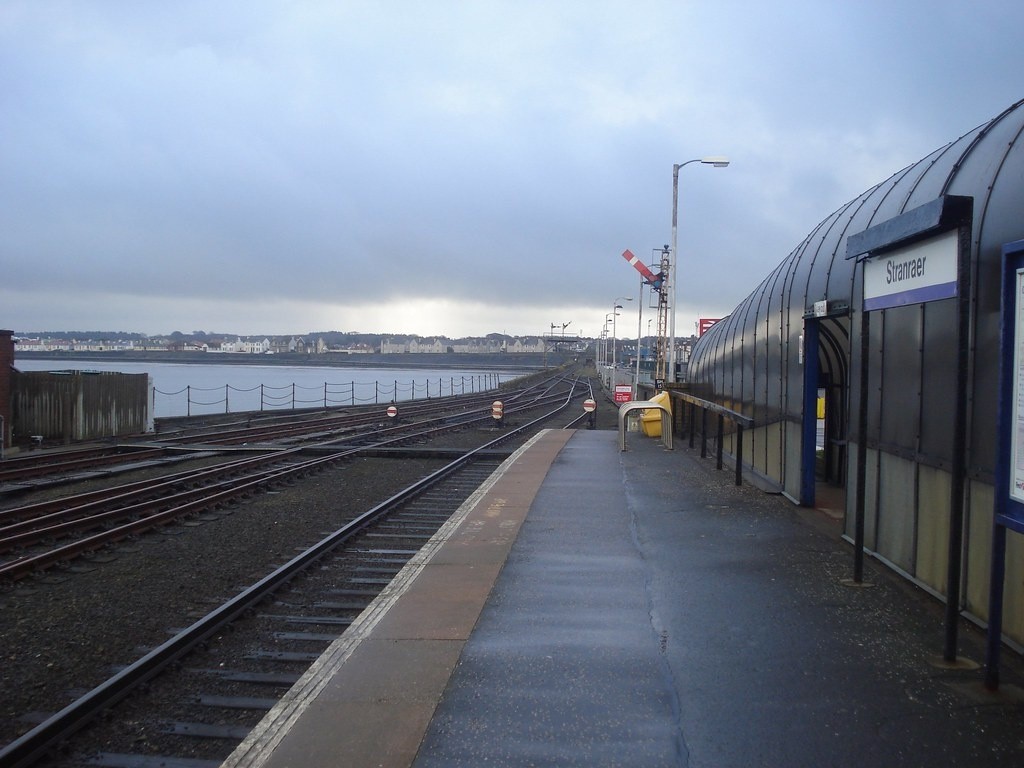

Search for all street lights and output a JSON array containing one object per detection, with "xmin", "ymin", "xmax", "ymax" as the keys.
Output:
[
  {"xmin": 634, "ymin": 263, "xmax": 662, "ymax": 402},
  {"xmin": 669, "ymin": 155, "xmax": 730, "ymax": 382},
  {"xmin": 597, "ymin": 296, "xmax": 633, "ymax": 392}
]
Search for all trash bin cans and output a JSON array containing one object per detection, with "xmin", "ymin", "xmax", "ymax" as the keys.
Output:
[{"xmin": 641, "ymin": 391, "xmax": 672, "ymax": 438}]
